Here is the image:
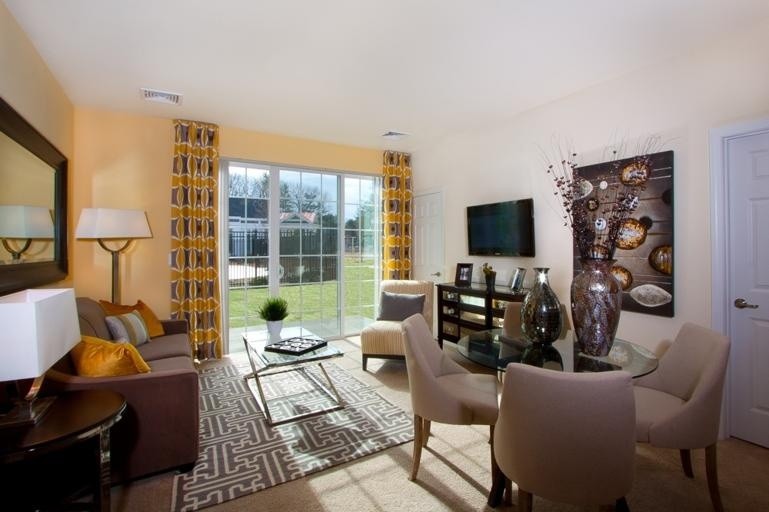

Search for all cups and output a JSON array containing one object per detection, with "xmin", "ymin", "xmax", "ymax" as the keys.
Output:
[
  {"xmin": 485, "ymin": 272, "xmax": 496, "ymax": 287},
  {"xmin": 487, "ymin": 288, "xmax": 496, "ymax": 293}
]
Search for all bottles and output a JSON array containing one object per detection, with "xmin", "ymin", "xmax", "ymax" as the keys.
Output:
[{"xmin": 520, "ymin": 345, "xmax": 564, "ymax": 371}]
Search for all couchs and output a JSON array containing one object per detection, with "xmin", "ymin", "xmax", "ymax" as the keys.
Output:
[{"xmin": 17, "ymin": 298, "xmax": 199, "ymax": 495}]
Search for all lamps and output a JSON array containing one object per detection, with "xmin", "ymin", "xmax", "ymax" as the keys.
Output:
[
  {"xmin": 73, "ymin": 209, "xmax": 154, "ymax": 304},
  {"xmin": 0, "ymin": 205, "xmax": 56, "ymax": 262},
  {"xmin": 0, "ymin": 286, "xmax": 81, "ymax": 429}
]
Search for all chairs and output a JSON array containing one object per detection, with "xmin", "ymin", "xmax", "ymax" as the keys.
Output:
[{"xmin": 361, "ymin": 279, "xmax": 435, "ymax": 370}]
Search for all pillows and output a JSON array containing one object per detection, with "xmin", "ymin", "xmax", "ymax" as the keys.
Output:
[
  {"xmin": 70, "ymin": 300, "xmax": 165, "ymax": 376},
  {"xmin": 376, "ymin": 290, "xmax": 426, "ymax": 321}
]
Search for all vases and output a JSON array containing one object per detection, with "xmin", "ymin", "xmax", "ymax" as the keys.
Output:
[{"xmin": 569, "ymin": 257, "xmax": 623, "ymax": 358}]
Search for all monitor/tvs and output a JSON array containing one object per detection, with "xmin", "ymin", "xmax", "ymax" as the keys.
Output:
[{"xmin": 467, "ymin": 198, "xmax": 535, "ymax": 256}]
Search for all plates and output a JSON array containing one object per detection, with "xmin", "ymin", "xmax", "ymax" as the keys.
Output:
[
  {"xmin": 620, "ymin": 164, "xmax": 653, "ymax": 186},
  {"xmin": 574, "ymin": 177, "xmax": 593, "ymax": 200},
  {"xmin": 609, "ymin": 219, "xmax": 648, "ymax": 249},
  {"xmin": 611, "ymin": 266, "xmax": 631, "ymax": 291},
  {"xmin": 629, "ymin": 283, "xmax": 671, "ymax": 307},
  {"xmin": 647, "ymin": 244, "xmax": 672, "ymax": 276}
]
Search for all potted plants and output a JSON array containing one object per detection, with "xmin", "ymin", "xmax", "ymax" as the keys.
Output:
[
  {"xmin": 259, "ymin": 296, "xmax": 289, "ymax": 334},
  {"xmin": 482, "ymin": 262, "xmax": 497, "ymax": 285}
]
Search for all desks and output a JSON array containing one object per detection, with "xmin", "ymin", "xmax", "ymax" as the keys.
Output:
[{"xmin": 0, "ymin": 387, "xmax": 126, "ymax": 511}]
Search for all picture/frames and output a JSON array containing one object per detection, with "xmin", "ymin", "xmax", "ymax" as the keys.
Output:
[
  {"xmin": 454, "ymin": 263, "xmax": 473, "ymax": 287},
  {"xmin": 511, "ymin": 267, "xmax": 527, "ymax": 292}
]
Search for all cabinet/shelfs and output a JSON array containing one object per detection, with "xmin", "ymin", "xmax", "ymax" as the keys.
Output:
[{"xmin": 435, "ymin": 281, "xmax": 532, "ymax": 350}]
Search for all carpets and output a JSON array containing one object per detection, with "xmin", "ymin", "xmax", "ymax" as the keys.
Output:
[{"xmin": 172, "ymin": 350, "xmax": 431, "ymax": 511}]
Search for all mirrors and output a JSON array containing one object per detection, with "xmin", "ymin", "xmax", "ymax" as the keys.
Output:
[{"xmin": 0, "ymin": 97, "xmax": 68, "ymax": 297}]
